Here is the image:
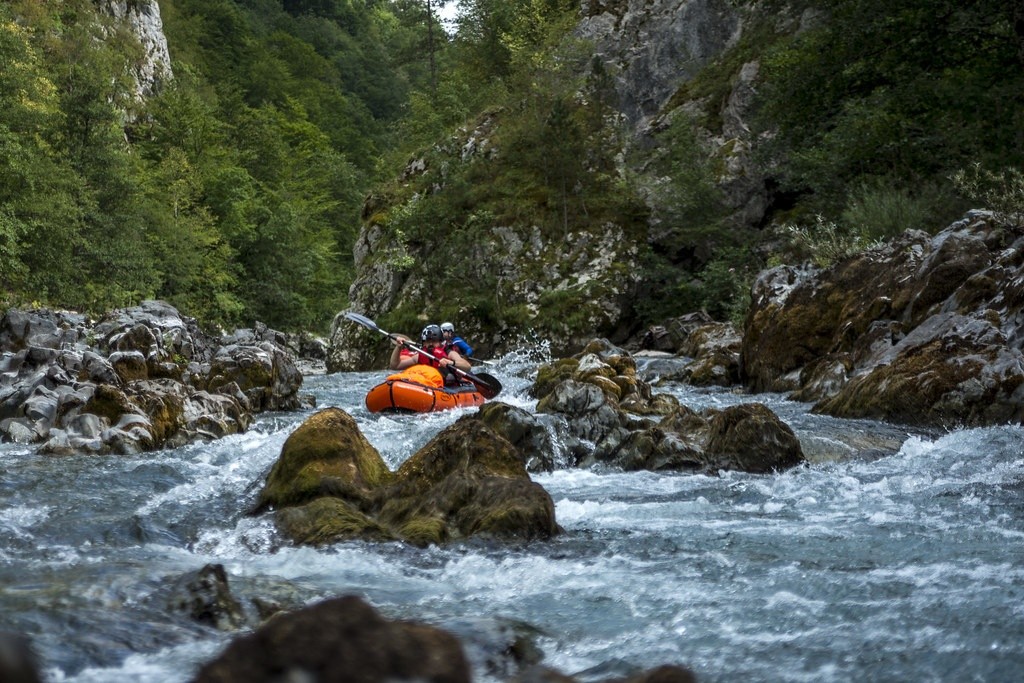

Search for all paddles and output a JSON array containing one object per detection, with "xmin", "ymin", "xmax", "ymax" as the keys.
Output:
[
  {"xmin": 345, "ymin": 313, "xmax": 503, "ymax": 400},
  {"xmin": 389, "ymin": 333, "xmax": 502, "ymax": 368}
]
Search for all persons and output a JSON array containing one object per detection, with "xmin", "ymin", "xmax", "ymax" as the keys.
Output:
[
  {"xmin": 441, "ymin": 322, "xmax": 473, "ymax": 358},
  {"xmin": 390, "ymin": 325, "xmax": 472, "ymax": 371}
]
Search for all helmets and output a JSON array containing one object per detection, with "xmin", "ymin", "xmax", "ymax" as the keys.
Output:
[
  {"xmin": 440, "ymin": 322, "xmax": 454, "ymax": 333},
  {"xmin": 421, "ymin": 322, "xmax": 444, "ymax": 342}
]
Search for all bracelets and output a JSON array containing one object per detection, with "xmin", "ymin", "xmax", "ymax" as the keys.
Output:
[{"xmin": 451, "ymin": 359, "xmax": 456, "ymax": 366}]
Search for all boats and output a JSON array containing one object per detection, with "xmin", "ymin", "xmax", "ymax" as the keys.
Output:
[{"xmin": 366, "ymin": 380, "xmax": 485, "ymax": 415}]
